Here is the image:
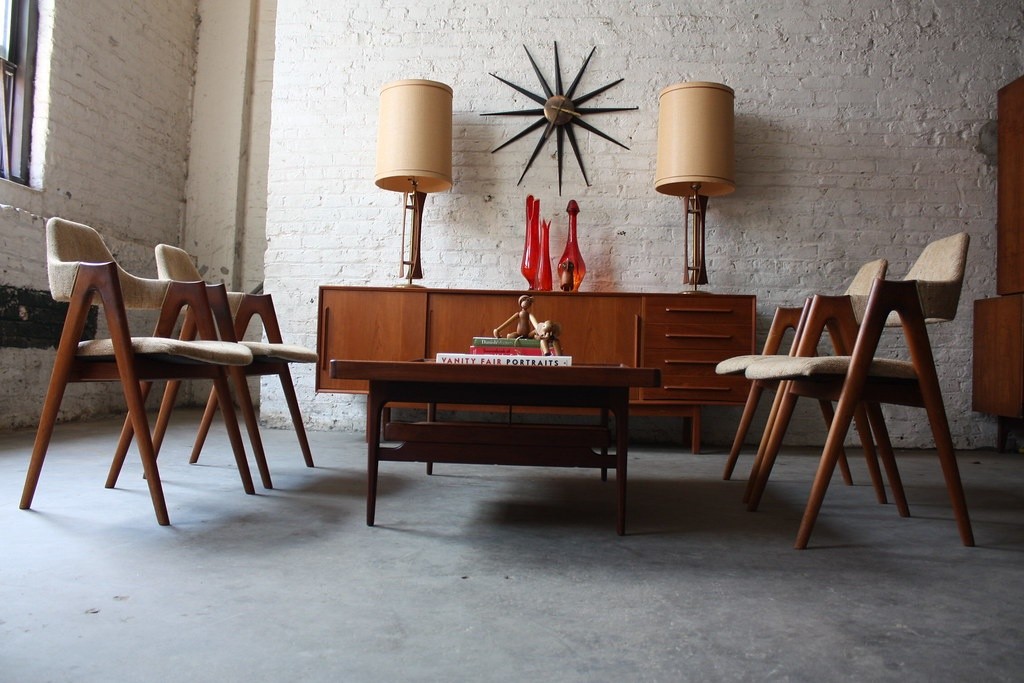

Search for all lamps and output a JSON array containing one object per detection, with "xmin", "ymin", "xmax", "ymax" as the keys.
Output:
[
  {"xmin": 654, "ymin": 81, "xmax": 737, "ymax": 294},
  {"xmin": 375, "ymin": 79, "xmax": 453, "ymax": 289}
]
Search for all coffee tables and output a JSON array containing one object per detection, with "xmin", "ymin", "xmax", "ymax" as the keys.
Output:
[{"xmin": 330, "ymin": 358, "xmax": 662, "ymax": 536}]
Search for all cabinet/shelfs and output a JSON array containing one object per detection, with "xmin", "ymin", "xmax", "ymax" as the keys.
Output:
[{"xmin": 315, "ymin": 285, "xmax": 756, "ymax": 454}]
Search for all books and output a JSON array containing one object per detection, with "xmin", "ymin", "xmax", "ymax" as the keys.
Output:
[{"xmin": 435, "ymin": 336, "xmax": 573, "ymax": 366}]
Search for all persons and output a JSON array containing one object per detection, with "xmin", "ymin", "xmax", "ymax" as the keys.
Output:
[
  {"xmin": 494, "ymin": 294, "xmax": 542, "ymax": 340},
  {"xmin": 538, "ymin": 320, "xmax": 561, "ymax": 356}
]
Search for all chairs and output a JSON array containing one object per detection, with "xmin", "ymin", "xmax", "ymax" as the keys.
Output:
[
  {"xmin": 143, "ymin": 241, "xmax": 320, "ymax": 490},
  {"xmin": 721, "ymin": 258, "xmax": 888, "ymax": 504},
  {"xmin": 19, "ymin": 216, "xmax": 256, "ymax": 527},
  {"xmin": 744, "ymin": 231, "xmax": 976, "ymax": 550}
]
